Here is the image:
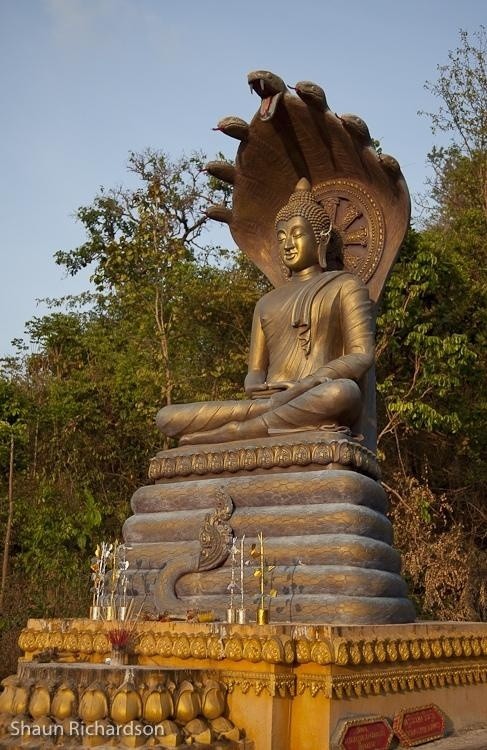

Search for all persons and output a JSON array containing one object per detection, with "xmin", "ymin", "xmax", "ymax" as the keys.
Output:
[{"xmin": 151, "ymin": 179, "xmax": 379, "ymax": 447}]
